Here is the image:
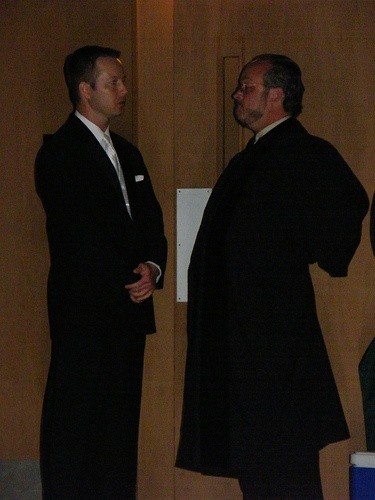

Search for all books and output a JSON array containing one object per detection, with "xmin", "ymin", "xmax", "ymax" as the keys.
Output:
[{"xmin": 349, "ymin": 451, "xmax": 375, "ymax": 500}]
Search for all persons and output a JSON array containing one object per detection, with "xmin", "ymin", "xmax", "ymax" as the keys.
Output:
[
  {"xmin": 33, "ymin": 45, "xmax": 170, "ymax": 500},
  {"xmin": 176, "ymin": 52, "xmax": 369, "ymax": 500}
]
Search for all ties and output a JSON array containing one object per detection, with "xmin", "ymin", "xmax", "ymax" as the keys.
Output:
[{"xmin": 99, "ymin": 135, "xmax": 134, "ymax": 224}]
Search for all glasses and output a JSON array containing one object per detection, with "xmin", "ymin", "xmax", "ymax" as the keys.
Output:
[{"xmin": 238, "ymin": 82, "xmax": 274, "ymax": 95}]
